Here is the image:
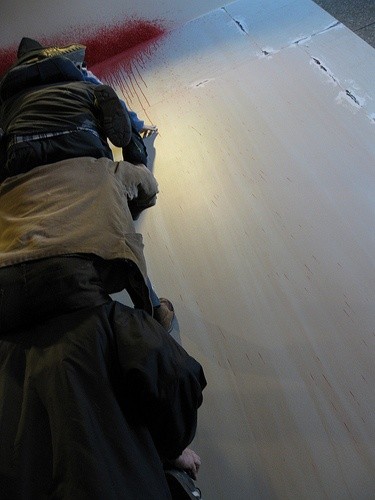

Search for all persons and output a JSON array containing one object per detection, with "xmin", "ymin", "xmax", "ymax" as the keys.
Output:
[
  {"xmin": 0, "ymin": 35, "xmax": 147, "ymax": 165},
  {"xmin": 0, "ymin": 301, "xmax": 208, "ymax": 500},
  {"xmin": 0, "ymin": 157, "xmax": 201, "ymax": 500},
  {"xmin": 0, "ymin": 78, "xmax": 175, "ymax": 334}
]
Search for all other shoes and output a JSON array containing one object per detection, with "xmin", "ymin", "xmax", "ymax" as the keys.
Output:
[
  {"xmin": 155, "ymin": 297, "xmax": 175, "ymax": 334},
  {"xmin": 94, "ymin": 85, "xmax": 132, "ymax": 147},
  {"xmin": 168, "ymin": 468, "xmax": 202, "ymax": 500}
]
